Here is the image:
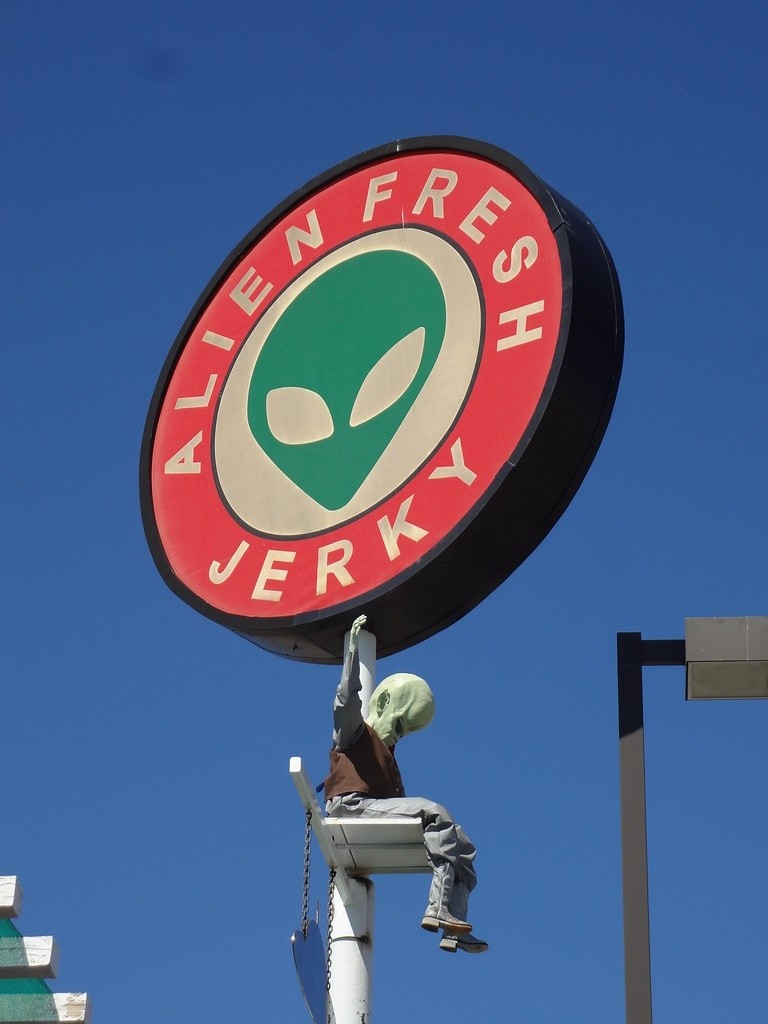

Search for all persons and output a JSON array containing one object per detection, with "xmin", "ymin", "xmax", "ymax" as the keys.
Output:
[{"xmin": 317, "ymin": 612, "xmax": 489, "ymax": 955}]
